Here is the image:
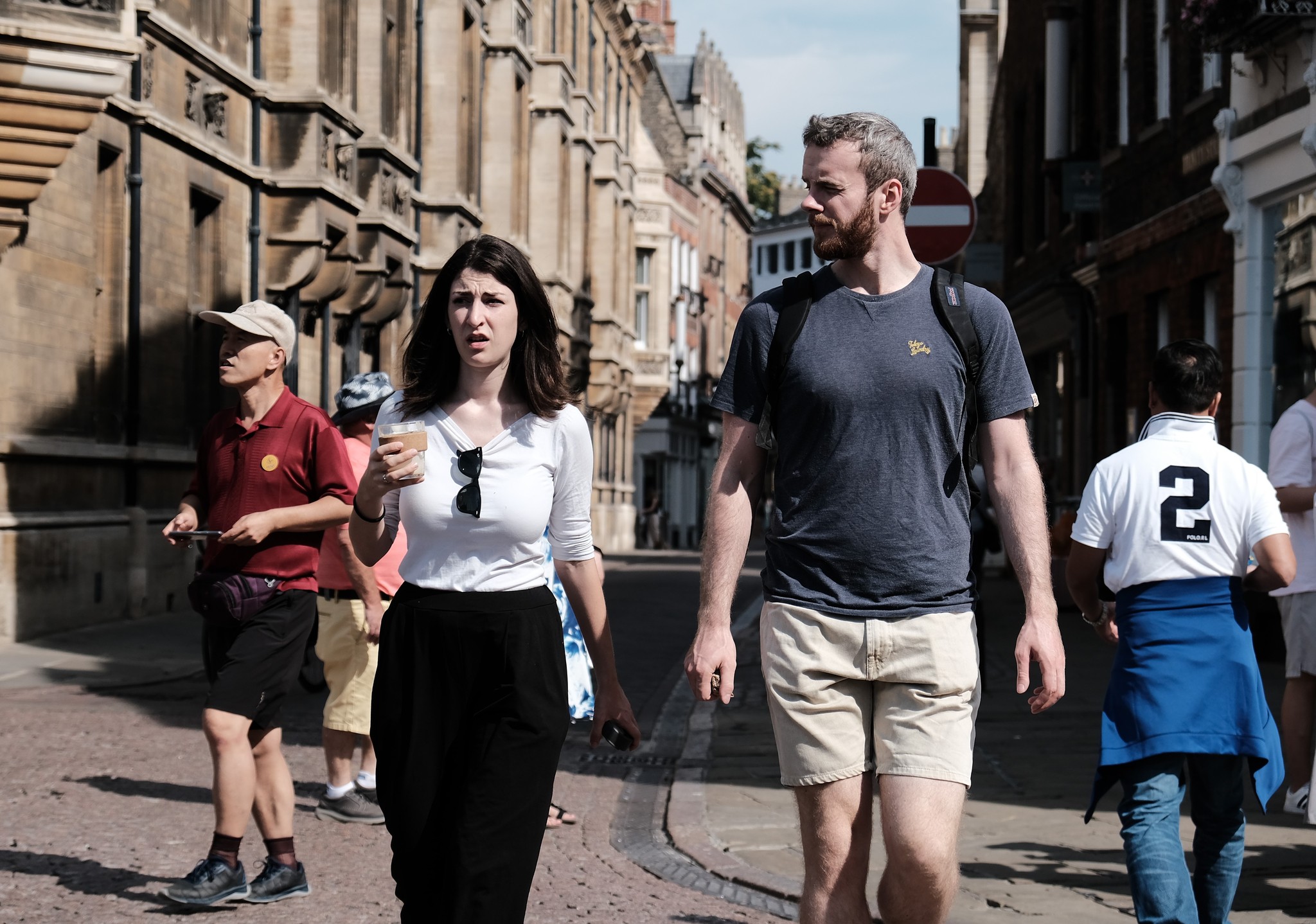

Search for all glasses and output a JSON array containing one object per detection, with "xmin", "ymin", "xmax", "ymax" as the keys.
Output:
[{"xmin": 456, "ymin": 447, "xmax": 483, "ymax": 518}]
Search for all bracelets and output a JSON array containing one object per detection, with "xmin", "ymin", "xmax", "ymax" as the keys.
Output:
[
  {"xmin": 353, "ymin": 494, "xmax": 386, "ymax": 523},
  {"xmin": 1081, "ymin": 600, "xmax": 1108, "ymax": 627}
]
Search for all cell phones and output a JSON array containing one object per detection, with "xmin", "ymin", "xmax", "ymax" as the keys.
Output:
[{"xmin": 169, "ymin": 530, "xmax": 224, "ymax": 540}]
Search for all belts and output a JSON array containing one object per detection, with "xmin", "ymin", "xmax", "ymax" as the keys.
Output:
[{"xmin": 317, "ymin": 586, "xmax": 390, "ymax": 602}]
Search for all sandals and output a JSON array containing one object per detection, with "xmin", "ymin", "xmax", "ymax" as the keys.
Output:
[{"xmin": 546, "ymin": 803, "xmax": 577, "ymax": 827}]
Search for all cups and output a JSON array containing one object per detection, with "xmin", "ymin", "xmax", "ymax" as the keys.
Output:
[{"xmin": 378, "ymin": 421, "xmax": 427, "ymax": 480}]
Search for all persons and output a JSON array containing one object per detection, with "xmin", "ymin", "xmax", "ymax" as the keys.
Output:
[
  {"xmin": 680, "ymin": 112, "xmax": 1065, "ymax": 924},
  {"xmin": 317, "ymin": 370, "xmax": 389, "ymax": 826},
  {"xmin": 538, "ymin": 522, "xmax": 606, "ymax": 829},
  {"xmin": 159, "ymin": 296, "xmax": 361, "ymax": 909},
  {"xmin": 347, "ymin": 233, "xmax": 641, "ymax": 924},
  {"xmin": 1269, "ymin": 377, "xmax": 1316, "ymax": 815},
  {"xmin": 1071, "ymin": 338, "xmax": 1296, "ymax": 924}
]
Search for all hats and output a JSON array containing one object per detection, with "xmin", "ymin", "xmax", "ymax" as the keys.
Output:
[
  {"xmin": 198, "ymin": 300, "xmax": 296, "ymax": 366},
  {"xmin": 331, "ymin": 371, "xmax": 395, "ymax": 426}
]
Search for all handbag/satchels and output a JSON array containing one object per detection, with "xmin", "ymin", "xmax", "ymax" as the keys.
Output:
[{"xmin": 187, "ymin": 571, "xmax": 282, "ymax": 621}]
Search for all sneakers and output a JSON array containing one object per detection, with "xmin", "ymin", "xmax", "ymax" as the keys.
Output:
[
  {"xmin": 243, "ymin": 862, "xmax": 311, "ymax": 903},
  {"xmin": 159, "ymin": 853, "xmax": 250, "ymax": 906},
  {"xmin": 316, "ymin": 780, "xmax": 385, "ymax": 823},
  {"xmin": 1283, "ymin": 782, "xmax": 1310, "ymax": 812}
]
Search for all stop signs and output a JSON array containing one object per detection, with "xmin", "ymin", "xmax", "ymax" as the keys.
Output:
[{"xmin": 903, "ymin": 167, "xmax": 978, "ymax": 267}]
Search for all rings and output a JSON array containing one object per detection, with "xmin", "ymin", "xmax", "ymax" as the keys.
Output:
[{"xmin": 383, "ymin": 472, "xmax": 392, "ymax": 484}]
